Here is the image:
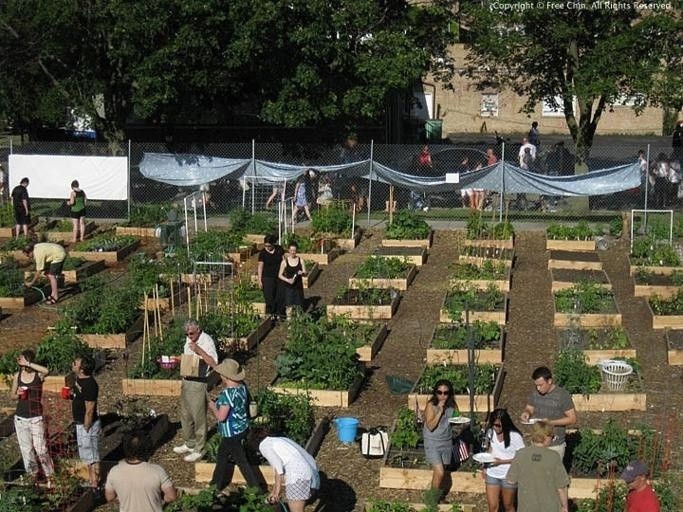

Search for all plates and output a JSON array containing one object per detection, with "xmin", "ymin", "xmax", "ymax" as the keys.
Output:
[
  {"xmin": 447, "ymin": 417, "xmax": 471, "ymax": 424},
  {"xmin": 520, "ymin": 418, "xmax": 542, "ymax": 426},
  {"xmin": 472, "ymin": 451, "xmax": 495, "ymax": 464}
]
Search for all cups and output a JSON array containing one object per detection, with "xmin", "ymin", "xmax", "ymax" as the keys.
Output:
[
  {"xmin": 19, "ymin": 386, "xmax": 29, "ymax": 401},
  {"xmin": 62, "ymin": 387, "xmax": 70, "ymax": 400}
]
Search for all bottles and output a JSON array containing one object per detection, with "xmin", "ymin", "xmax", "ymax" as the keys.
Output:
[{"xmin": 292, "ymin": 273, "xmax": 298, "ymax": 281}]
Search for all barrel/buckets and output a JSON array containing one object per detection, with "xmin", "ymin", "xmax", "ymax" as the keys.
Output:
[{"xmin": 335, "ymin": 417, "xmax": 359, "ymax": 442}]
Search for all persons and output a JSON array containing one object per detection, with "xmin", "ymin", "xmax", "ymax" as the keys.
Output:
[
  {"xmin": 11, "ymin": 178, "xmax": 31, "ymax": 241},
  {"xmin": 173, "ymin": 319, "xmax": 218, "ymax": 462},
  {"xmin": 636, "ymin": 149, "xmax": 683, "ymax": 208},
  {"xmin": 423, "ymin": 379, "xmax": 455, "ymax": 504},
  {"xmin": 456, "ymin": 147, "xmax": 498, "ymax": 212},
  {"xmin": 66, "ymin": 180, "xmax": 87, "ymax": 243},
  {"xmin": 620, "ymin": 460, "xmax": 660, "ymax": 512},
  {"xmin": 519, "ymin": 122, "xmax": 590, "ymax": 176},
  {"xmin": 105, "ymin": 431, "xmax": 176, "ymax": 512},
  {"xmin": 250, "ymin": 434, "xmax": 321, "ymax": 512},
  {"xmin": 278, "ymin": 241, "xmax": 306, "ymax": 306},
  {"xmin": 10, "ymin": 350, "xmax": 55, "ymax": 479},
  {"xmin": 258, "ymin": 236, "xmax": 285, "ymax": 315},
  {"xmin": 506, "ymin": 421, "xmax": 570, "ymax": 512},
  {"xmin": 69, "ymin": 355, "xmax": 102, "ymax": 488},
  {"xmin": 520, "ymin": 367, "xmax": 577, "ymax": 462},
  {"xmin": 419, "ymin": 145, "xmax": 433, "ymax": 177},
  {"xmin": 23, "ymin": 243, "xmax": 66, "ymax": 305},
  {"xmin": 208, "ymin": 359, "xmax": 267, "ymax": 493},
  {"xmin": 481, "ymin": 408, "xmax": 525, "ymax": 512},
  {"xmin": 267, "ymin": 171, "xmax": 332, "ymax": 222}
]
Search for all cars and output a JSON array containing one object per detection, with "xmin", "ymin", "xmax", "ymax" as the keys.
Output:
[{"xmin": 404, "ymin": 148, "xmax": 517, "ymax": 199}]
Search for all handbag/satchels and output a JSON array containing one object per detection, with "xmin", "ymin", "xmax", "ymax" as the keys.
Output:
[
  {"xmin": 361, "ymin": 425, "xmax": 390, "ymax": 459},
  {"xmin": 452, "ymin": 439, "xmax": 470, "ymax": 464}
]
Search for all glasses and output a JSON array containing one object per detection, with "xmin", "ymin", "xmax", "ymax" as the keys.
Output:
[
  {"xmin": 184, "ymin": 331, "xmax": 196, "ymax": 336},
  {"xmin": 490, "ymin": 422, "xmax": 502, "ymax": 428},
  {"xmin": 435, "ymin": 389, "xmax": 450, "ymax": 396},
  {"xmin": 546, "ymin": 434, "xmax": 555, "ymax": 439}
]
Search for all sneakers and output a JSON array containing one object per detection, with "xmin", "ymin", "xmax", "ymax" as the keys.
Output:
[
  {"xmin": 172, "ymin": 444, "xmax": 195, "ymax": 454},
  {"xmin": 183, "ymin": 452, "xmax": 202, "ymax": 463}
]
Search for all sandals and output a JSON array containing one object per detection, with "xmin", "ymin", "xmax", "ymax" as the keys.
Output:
[{"xmin": 45, "ymin": 295, "xmax": 57, "ymax": 305}]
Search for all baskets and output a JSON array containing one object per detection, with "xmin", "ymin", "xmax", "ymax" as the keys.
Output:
[{"xmin": 599, "ymin": 359, "xmax": 634, "ymax": 391}]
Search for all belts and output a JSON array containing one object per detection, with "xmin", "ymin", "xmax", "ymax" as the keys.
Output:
[{"xmin": 184, "ymin": 375, "xmax": 208, "ymax": 383}]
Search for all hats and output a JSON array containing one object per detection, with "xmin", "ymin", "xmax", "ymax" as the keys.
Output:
[
  {"xmin": 214, "ymin": 358, "xmax": 246, "ymax": 382},
  {"xmin": 621, "ymin": 460, "xmax": 648, "ymax": 482}
]
